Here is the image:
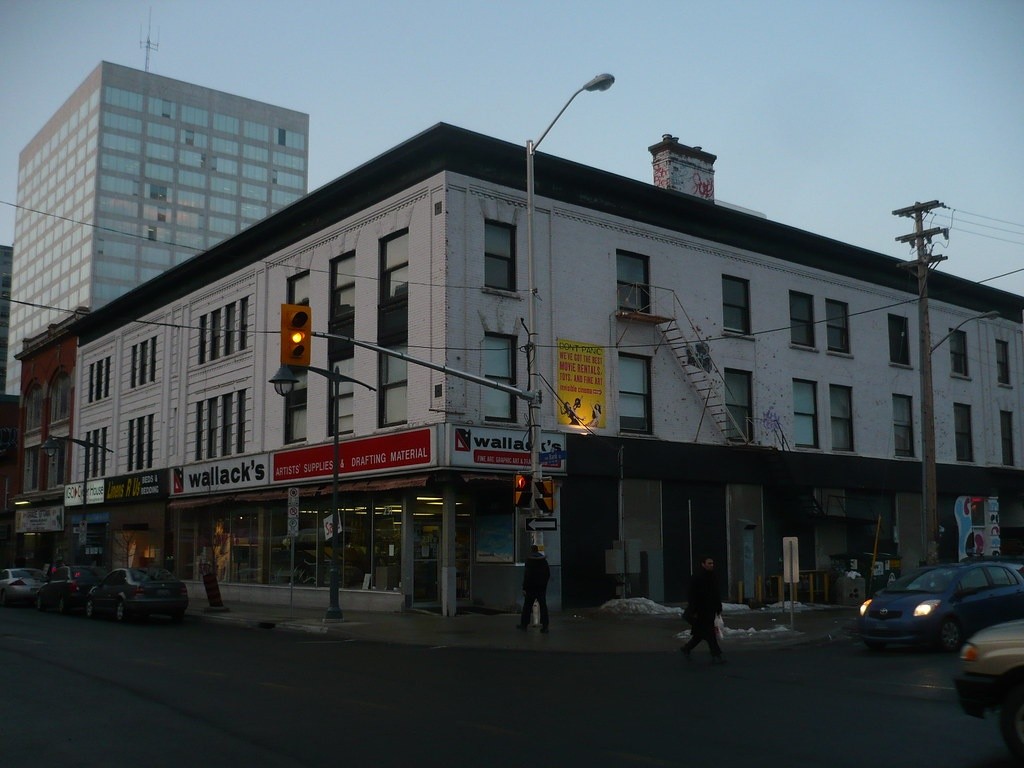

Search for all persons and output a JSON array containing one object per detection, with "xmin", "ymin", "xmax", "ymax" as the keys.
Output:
[
  {"xmin": 682, "ymin": 556, "xmax": 727, "ymax": 665},
  {"xmin": 517, "ymin": 545, "xmax": 550, "ymax": 634}
]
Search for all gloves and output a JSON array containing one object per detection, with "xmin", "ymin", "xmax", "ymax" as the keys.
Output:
[{"xmin": 714, "ymin": 604, "xmax": 722, "ymax": 615}]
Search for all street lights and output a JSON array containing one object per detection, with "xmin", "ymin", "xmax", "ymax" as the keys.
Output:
[{"xmin": 526, "ymin": 71, "xmax": 618, "ymax": 628}]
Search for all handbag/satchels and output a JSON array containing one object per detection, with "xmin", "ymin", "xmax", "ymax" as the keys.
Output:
[
  {"xmin": 713, "ymin": 612, "xmax": 724, "ymax": 640},
  {"xmin": 682, "ymin": 606, "xmax": 703, "ymax": 627}
]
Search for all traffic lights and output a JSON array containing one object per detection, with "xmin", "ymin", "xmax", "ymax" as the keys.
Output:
[
  {"xmin": 280, "ymin": 303, "xmax": 312, "ymax": 367},
  {"xmin": 534, "ymin": 480, "xmax": 557, "ymax": 514},
  {"xmin": 513, "ymin": 473, "xmax": 530, "ymax": 507}
]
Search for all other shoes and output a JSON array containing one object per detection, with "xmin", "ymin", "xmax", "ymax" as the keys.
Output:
[
  {"xmin": 681, "ymin": 647, "xmax": 692, "ymax": 657},
  {"xmin": 711, "ymin": 656, "xmax": 727, "ymax": 663},
  {"xmin": 540, "ymin": 628, "xmax": 549, "ymax": 633},
  {"xmin": 516, "ymin": 624, "xmax": 528, "ymax": 631}
]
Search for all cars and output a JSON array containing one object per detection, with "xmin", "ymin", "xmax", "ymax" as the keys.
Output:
[
  {"xmin": 0, "ymin": 567, "xmax": 46, "ymax": 605},
  {"xmin": 951, "ymin": 617, "xmax": 1024, "ymax": 762},
  {"xmin": 957, "ymin": 554, "xmax": 1024, "ymax": 580},
  {"xmin": 85, "ymin": 566, "xmax": 190, "ymax": 622},
  {"xmin": 37, "ymin": 565, "xmax": 110, "ymax": 615},
  {"xmin": 853, "ymin": 561, "xmax": 1024, "ymax": 651}
]
